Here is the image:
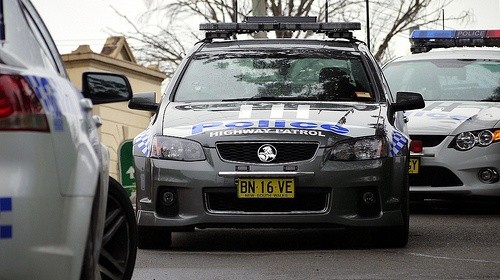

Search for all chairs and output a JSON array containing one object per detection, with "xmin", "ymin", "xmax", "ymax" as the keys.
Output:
[
  {"xmin": 404, "ymin": 70, "xmax": 440, "ymax": 101},
  {"xmin": 316, "ymin": 66, "xmax": 356, "ymax": 101}
]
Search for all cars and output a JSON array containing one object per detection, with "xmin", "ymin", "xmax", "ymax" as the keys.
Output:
[
  {"xmin": 127, "ymin": 16, "xmax": 424, "ymax": 250},
  {"xmin": 0, "ymin": 1, "xmax": 139, "ymax": 280},
  {"xmin": 380, "ymin": 28, "xmax": 500, "ymax": 213}
]
